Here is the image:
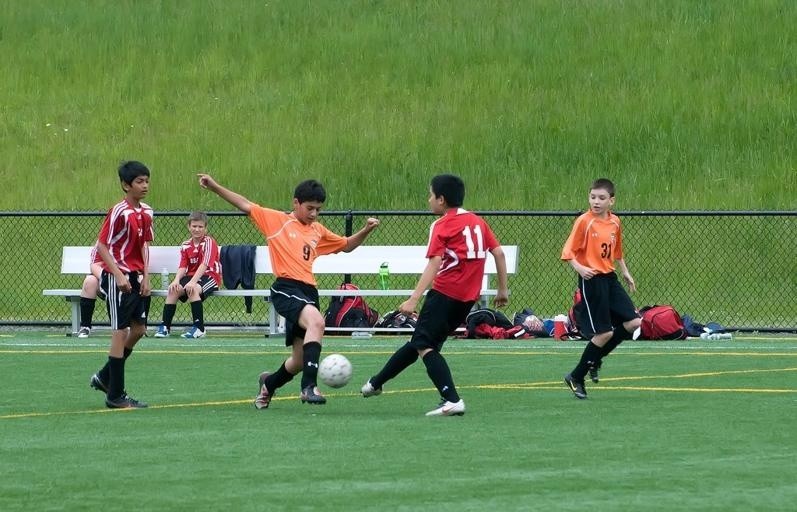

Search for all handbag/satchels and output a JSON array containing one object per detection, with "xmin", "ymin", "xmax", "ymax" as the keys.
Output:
[
  {"xmin": 324, "ymin": 284, "xmax": 378, "ymax": 335},
  {"xmin": 641, "ymin": 306, "xmax": 687, "ymax": 340},
  {"xmin": 374, "ymin": 309, "xmax": 417, "ymax": 334},
  {"xmin": 466, "ymin": 309, "xmax": 512, "ymax": 339}
]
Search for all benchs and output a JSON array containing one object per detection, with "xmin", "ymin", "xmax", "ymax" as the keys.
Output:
[{"xmin": 40, "ymin": 245, "xmax": 520, "ymax": 339}]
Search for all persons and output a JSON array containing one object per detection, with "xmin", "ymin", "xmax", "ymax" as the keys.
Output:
[
  {"xmin": 153, "ymin": 210, "xmax": 224, "ymax": 341},
  {"xmin": 196, "ymin": 171, "xmax": 380, "ymax": 412},
  {"xmin": 76, "ymin": 244, "xmax": 151, "ymax": 339},
  {"xmin": 359, "ymin": 172, "xmax": 510, "ymax": 417},
  {"xmin": 558, "ymin": 177, "xmax": 642, "ymax": 401},
  {"xmin": 88, "ymin": 159, "xmax": 155, "ymax": 411}
]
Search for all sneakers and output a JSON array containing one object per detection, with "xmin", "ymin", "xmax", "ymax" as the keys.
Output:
[
  {"xmin": 180, "ymin": 326, "xmax": 206, "ymax": 338},
  {"xmin": 78, "ymin": 327, "xmax": 89, "ymax": 339},
  {"xmin": 565, "ymin": 372, "xmax": 586, "ymax": 398},
  {"xmin": 588, "ymin": 367, "xmax": 600, "ymax": 383},
  {"xmin": 89, "ymin": 372, "xmax": 146, "ymax": 409},
  {"xmin": 426, "ymin": 399, "xmax": 465, "ymax": 416},
  {"xmin": 361, "ymin": 378, "xmax": 384, "ymax": 398},
  {"xmin": 300, "ymin": 383, "xmax": 326, "ymax": 403},
  {"xmin": 254, "ymin": 372, "xmax": 273, "ymax": 409},
  {"xmin": 155, "ymin": 326, "xmax": 169, "ymax": 338}
]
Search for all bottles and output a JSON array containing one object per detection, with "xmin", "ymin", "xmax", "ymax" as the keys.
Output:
[
  {"xmin": 161, "ymin": 267, "xmax": 170, "ymax": 289},
  {"xmin": 379, "ymin": 262, "xmax": 390, "ymax": 290},
  {"xmin": 352, "ymin": 331, "xmax": 372, "ymax": 340},
  {"xmin": 700, "ymin": 332, "xmax": 732, "ymax": 341}
]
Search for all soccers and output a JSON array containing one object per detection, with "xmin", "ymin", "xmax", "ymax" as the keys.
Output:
[{"xmin": 319, "ymin": 354, "xmax": 352, "ymax": 388}]
